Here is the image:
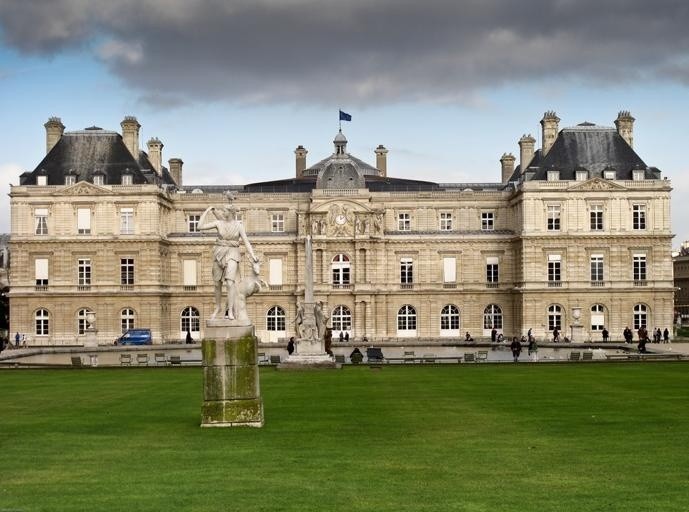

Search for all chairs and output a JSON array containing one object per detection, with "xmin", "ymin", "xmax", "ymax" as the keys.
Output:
[
  {"xmin": 257, "ymin": 353, "xmax": 281, "ymax": 366},
  {"xmin": 71, "ymin": 356, "xmax": 88, "ymax": 368},
  {"xmin": 335, "ymin": 348, "xmax": 438, "ymax": 368},
  {"xmin": 464, "ymin": 351, "xmax": 488, "ymax": 363},
  {"xmin": 118, "ymin": 352, "xmax": 182, "ymax": 368},
  {"xmin": 567, "ymin": 350, "xmax": 593, "ymax": 361}
]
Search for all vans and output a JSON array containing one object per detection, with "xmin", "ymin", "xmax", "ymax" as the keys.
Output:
[{"xmin": 114, "ymin": 329, "xmax": 153, "ymax": 346}]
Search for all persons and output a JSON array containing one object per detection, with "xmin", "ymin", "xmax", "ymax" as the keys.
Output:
[
  {"xmin": 14, "ymin": 331, "xmax": 20, "ymax": 350},
  {"xmin": 464, "ymin": 334, "xmax": 473, "ymax": 341},
  {"xmin": 186, "ymin": 328, "xmax": 193, "ymax": 343},
  {"xmin": 197, "ymin": 204, "xmax": 258, "ymax": 320},
  {"xmin": 491, "ymin": 326, "xmax": 538, "ymax": 366},
  {"xmin": 315, "ymin": 301, "xmax": 324, "ymax": 338},
  {"xmin": 339, "ymin": 331, "xmax": 343, "ymax": 341},
  {"xmin": 290, "ymin": 303, "xmax": 304, "ymax": 338},
  {"xmin": 623, "ymin": 326, "xmax": 669, "ymax": 354},
  {"xmin": 602, "ymin": 328, "xmax": 609, "ymax": 342},
  {"xmin": 344, "ymin": 331, "xmax": 349, "ymax": 341},
  {"xmin": 553, "ymin": 325, "xmax": 560, "ymax": 343},
  {"xmin": 286, "ymin": 337, "xmax": 295, "ymax": 355},
  {"xmin": 22, "ymin": 334, "xmax": 28, "ymax": 349}
]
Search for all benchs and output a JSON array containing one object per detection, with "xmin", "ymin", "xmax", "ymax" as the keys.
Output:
[
  {"xmin": 606, "ymin": 353, "xmax": 683, "ymax": 361},
  {"xmin": 0, "ymin": 361, "xmax": 20, "ymax": 368}
]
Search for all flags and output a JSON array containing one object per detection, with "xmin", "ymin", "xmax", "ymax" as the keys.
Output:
[{"xmin": 339, "ymin": 110, "xmax": 352, "ymax": 121}]
